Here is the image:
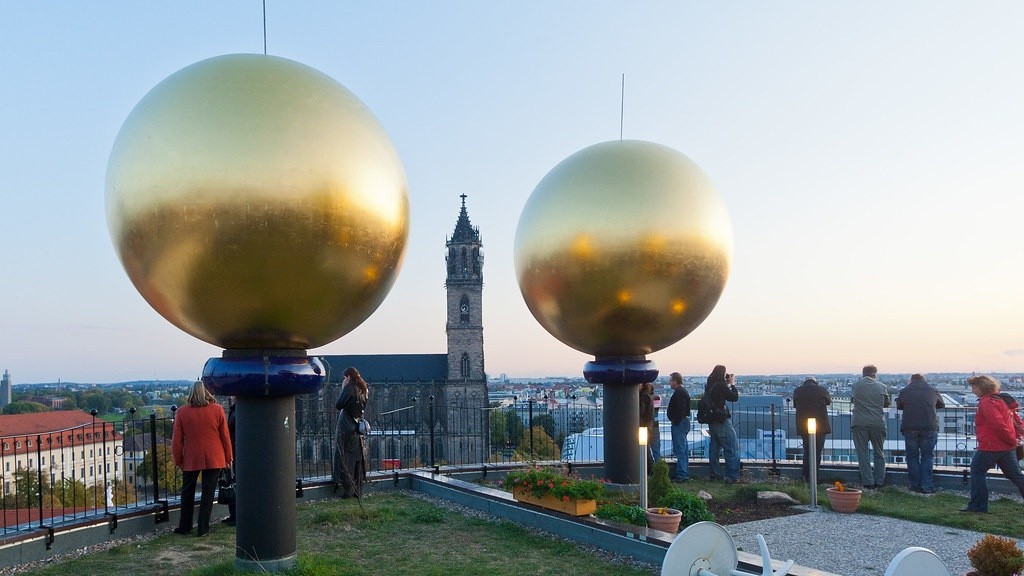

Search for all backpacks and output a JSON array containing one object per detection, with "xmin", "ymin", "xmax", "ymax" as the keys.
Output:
[{"xmin": 697, "ymin": 382, "xmax": 725, "ymax": 424}]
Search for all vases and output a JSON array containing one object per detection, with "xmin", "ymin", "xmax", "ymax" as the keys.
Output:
[
  {"xmin": 645, "ymin": 508, "xmax": 682, "ymax": 534},
  {"xmin": 964, "ymin": 571, "xmax": 977, "ymax": 576},
  {"xmin": 826, "ymin": 487, "xmax": 865, "ymax": 513},
  {"xmin": 507, "ymin": 487, "xmax": 602, "ymax": 515}
]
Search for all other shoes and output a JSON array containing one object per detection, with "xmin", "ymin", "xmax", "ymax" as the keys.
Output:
[
  {"xmin": 173, "ymin": 528, "xmax": 193, "ymax": 535},
  {"xmin": 710, "ymin": 476, "xmax": 726, "ymax": 482},
  {"xmin": 199, "ymin": 527, "xmax": 212, "ymax": 536},
  {"xmin": 352, "ymin": 492, "xmax": 360, "ymax": 497},
  {"xmin": 671, "ymin": 476, "xmax": 690, "ymax": 483},
  {"xmin": 221, "ymin": 515, "xmax": 236, "ymax": 526},
  {"xmin": 908, "ymin": 487, "xmax": 934, "ymax": 494},
  {"xmin": 725, "ymin": 477, "xmax": 745, "ymax": 483},
  {"xmin": 862, "ymin": 483, "xmax": 882, "ymax": 489},
  {"xmin": 958, "ymin": 507, "xmax": 968, "ymax": 512},
  {"xmin": 341, "ymin": 493, "xmax": 351, "ymax": 499}
]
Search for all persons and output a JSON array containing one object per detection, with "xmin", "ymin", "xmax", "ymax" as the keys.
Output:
[
  {"xmin": 897, "ymin": 374, "xmax": 945, "ymax": 493},
  {"xmin": 332, "ymin": 367, "xmax": 368, "ymax": 498},
  {"xmin": 638, "ymin": 383, "xmax": 661, "ymax": 477},
  {"xmin": 705, "ymin": 365, "xmax": 745, "ymax": 483},
  {"xmin": 957, "ymin": 376, "xmax": 1024, "ymax": 512},
  {"xmin": 793, "ymin": 376, "xmax": 831, "ymax": 482},
  {"xmin": 850, "ymin": 365, "xmax": 890, "ymax": 488},
  {"xmin": 171, "ymin": 381, "xmax": 233, "ymax": 536},
  {"xmin": 220, "ymin": 396, "xmax": 237, "ymax": 527},
  {"xmin": 667, "ymin": 372, "xmax": 691, "ymax": 483}
]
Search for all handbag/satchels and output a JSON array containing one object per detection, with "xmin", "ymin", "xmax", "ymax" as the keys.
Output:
[
  {"xmin": 358, "ymin": 418, "xmax": 371, "ymax": 435},
  {"xmin": 218, "ymin": 466, "xmax": 237, "ymax": 504}
]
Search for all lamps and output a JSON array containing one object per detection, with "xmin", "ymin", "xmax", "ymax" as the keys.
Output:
[
  {"xmin": 808, "ymin": 418, "xmax": 817, "ymax": 435},
  {"xmin": 639, "ymin": 426, "xmax": 649, "ymax": 445}
]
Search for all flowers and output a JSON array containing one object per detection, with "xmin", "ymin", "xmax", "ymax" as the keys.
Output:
[
  {"xmin": 966, "ymin": 533, "xmax": 1024, "ymax": 576},
  {"xmin": 657, "ymin": 507, "xmax": 669, "ymax": 515},
  {"xmin": 497, "ymin": 465, "xmax": 606, "ymax": 501},
  {"xmin": 834, "ymin": 480, "xmax": 858, "ymax": 491}
]
